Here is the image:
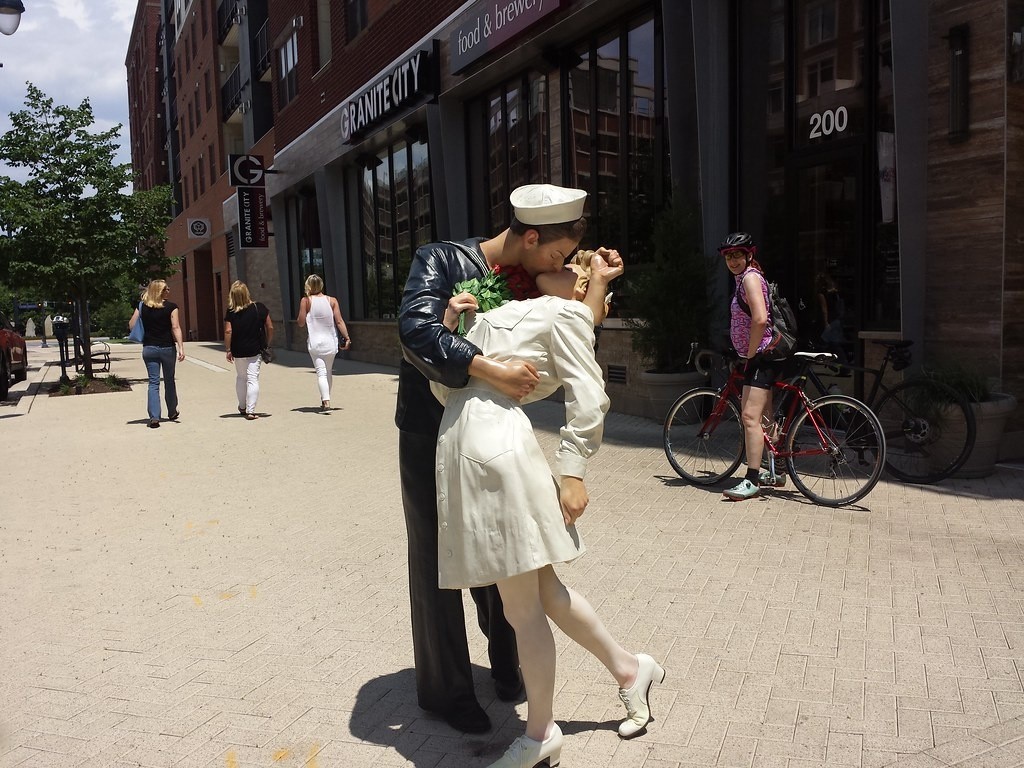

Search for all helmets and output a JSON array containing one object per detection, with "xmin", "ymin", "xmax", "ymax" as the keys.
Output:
[{"xmin": 717, "ymin": 232, "xmax": 757, "ymax": 256}]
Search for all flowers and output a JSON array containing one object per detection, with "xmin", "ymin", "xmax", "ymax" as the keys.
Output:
[{"xmin": 451, "ymin": 262, "xmax": 540, "ymax": 337}]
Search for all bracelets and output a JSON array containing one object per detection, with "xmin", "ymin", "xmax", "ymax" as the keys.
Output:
[{"xmin": 346, "ymin": 337, "xmax": 350, "ymax": 340}]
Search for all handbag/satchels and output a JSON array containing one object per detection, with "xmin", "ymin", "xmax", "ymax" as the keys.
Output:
[
  {"xmin": 129, "ymin": 301, "xmax": 144, "ymax": 342},
  {"xmin": 260, "ymin": 347, "xmax": 275, "ymax": 364}
]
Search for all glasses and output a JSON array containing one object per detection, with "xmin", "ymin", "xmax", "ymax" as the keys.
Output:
[{"xmin": 725, "ymin": 251, "xmax": 745, "ymax": 259}]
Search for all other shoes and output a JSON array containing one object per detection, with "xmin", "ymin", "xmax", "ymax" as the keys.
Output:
[{"xmin": 319, "ymin": 407, "xmax": 332, "ymax": 413}]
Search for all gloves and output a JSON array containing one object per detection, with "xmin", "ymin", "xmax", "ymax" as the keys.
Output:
[{"xmin": 747, "ymin": 357, "xmax": 760, "ymax": 370}]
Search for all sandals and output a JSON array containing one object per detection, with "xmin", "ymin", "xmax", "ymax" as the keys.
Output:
[
  {"xmin": 147, "ymin": 421, "xmax": 160, "ymax": 428},
  {"xmin": 244, "ymin": 414, "xmax": 255, "ymax": 419},
  {"xmin": 169, "ymin": 412, "xmax": 179, "ymax": 419},
  {"xmin": 238, "ymin": 406, "xmax": 246, "ymax": 412}
]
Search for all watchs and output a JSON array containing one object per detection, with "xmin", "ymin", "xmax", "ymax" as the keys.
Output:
[{"xmin": 718, "ymin": 232, "xmax": 786, "ymax": 501}]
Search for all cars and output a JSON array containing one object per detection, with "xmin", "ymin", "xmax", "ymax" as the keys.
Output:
[{"xmin": 0, "ymin": 310, "xmax": 28, "ymax": 401}]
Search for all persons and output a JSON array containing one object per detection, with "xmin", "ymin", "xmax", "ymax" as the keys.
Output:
[
  {"xmin": 296, "ymin": 274, "xmax": 351, "ymax": 413},
  {"xmin": 129, "ymin": 279, "xmax": 185, "ymax": 428},
  {"xmin": 395, "ymin": 184, "xmax": 665, "ymax": 768},
  {"xmin": 225, "ymin": 280, "xmax": 273, "ymax": 419},
  {"xmin": 816, "ymin": 270, "xmax": 848, "ymax": 370}
]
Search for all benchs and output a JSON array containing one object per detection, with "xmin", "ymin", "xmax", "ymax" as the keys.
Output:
[{"xmin": 76, "ymin": 335, "xmax": 111, "ymax": 374}]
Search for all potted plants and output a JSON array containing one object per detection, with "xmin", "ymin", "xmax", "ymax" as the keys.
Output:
[{"xmin": 613, "ymin": 192, "xmax": 726, "ymax": 426}]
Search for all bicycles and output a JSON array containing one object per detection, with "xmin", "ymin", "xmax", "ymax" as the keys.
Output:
[
  {"xmin": 761, "ymin": 337, "xmax": 976, "ymax": 484},
  {"xmin": 663, "ymin": 338, "xmax": 887, "ymax": 509}
]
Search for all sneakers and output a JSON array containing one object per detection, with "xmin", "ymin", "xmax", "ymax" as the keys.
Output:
[
  {"xmin": 759, "ymin": 471, "xmax": 786, "ymax": 487},
  {"xmin": 723, "ymin": 479, "xmax": 762, "ymax": 501}
]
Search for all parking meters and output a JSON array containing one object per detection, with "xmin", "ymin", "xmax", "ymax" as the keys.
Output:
[{"xmin": 52, "ymin": 316, "xmax": 70, "ymax": 382}]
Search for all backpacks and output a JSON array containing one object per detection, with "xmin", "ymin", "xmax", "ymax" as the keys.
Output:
[{"xmin": 737, "ymin": 269, "xmax": 798, "ymax": 370}]
